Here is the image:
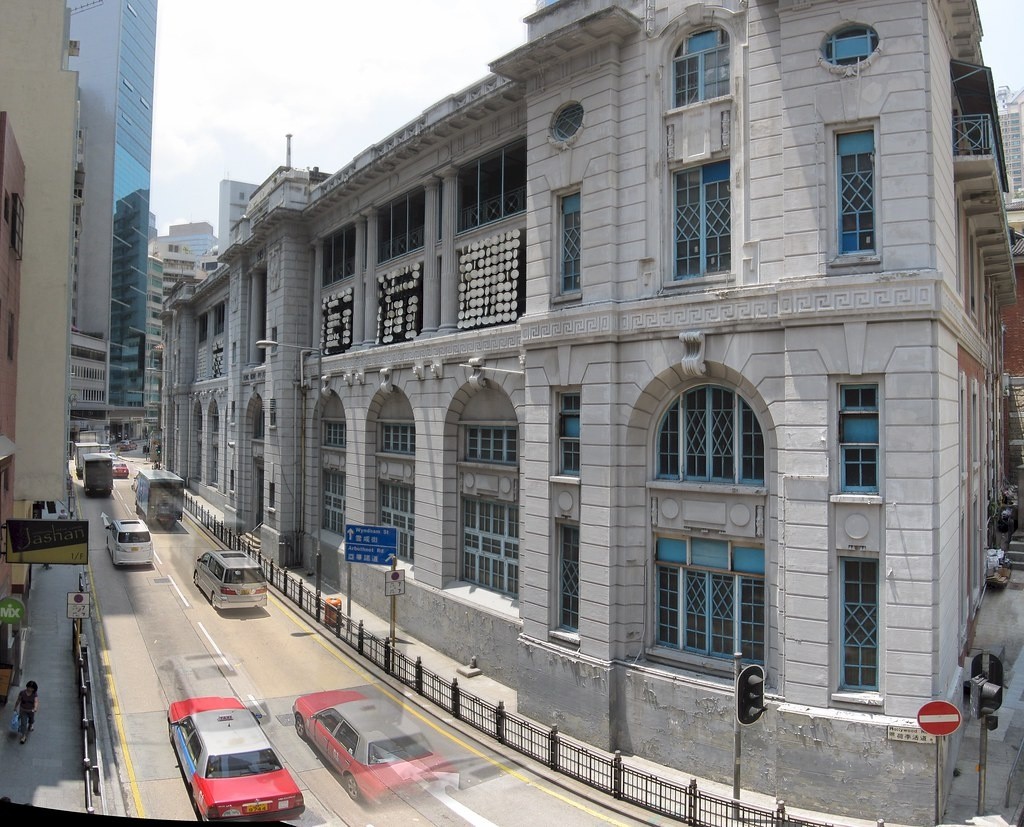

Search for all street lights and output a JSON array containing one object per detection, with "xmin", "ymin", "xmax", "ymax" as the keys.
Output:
[
  {"xmin": 147, "ymin": 367, "xmax": 175, "ymax": 471},
  {"xmin": 256, "ymin": 340, "xmax": 324, "ymax": 596}
]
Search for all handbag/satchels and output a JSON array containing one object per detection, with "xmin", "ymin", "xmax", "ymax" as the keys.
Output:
[{"xmin": 12, "ymin": 711, "xmax": 20, "ymax": 730}]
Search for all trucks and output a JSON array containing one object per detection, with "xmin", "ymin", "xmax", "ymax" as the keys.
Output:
[
  {"xmin": 74, "ymin": 442, "xmax": 101, "ymax": 480},
  {"xmin": 131, "ymin": 468, "xmax": 185, "ymax": 528},
  {"xmin": 79, "ymin": 429, "xmax": 108, "ymax": 445},
  {"xmin": 80, "ymin": 452, "xmax": 114, "ymax": 497}
]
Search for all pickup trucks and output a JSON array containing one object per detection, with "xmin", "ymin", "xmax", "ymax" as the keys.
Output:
[{"xmin": 116, "ymin": 439, "xmax": 138, "ymax": 451}]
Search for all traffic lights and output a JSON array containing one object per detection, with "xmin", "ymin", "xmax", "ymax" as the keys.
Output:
[
  {"xmin": 736, "ymin": 664, "xmax": 766, "ymax": 726},
  {"xmin": 971, "ymin": 675, "xmax": 1002, "ymax": 718},
  {"xmin": 157, "ymin": 445, "xmax": 162, "ymax": 455}
]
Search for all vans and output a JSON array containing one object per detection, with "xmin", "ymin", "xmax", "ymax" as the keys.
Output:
[
  {"xmin": 193, "ymin": 550, "xmax": 268, "ymax": 611},
  {"xmin": 105, "ymin": 519, "xmax": 154, "ymax": 569}
]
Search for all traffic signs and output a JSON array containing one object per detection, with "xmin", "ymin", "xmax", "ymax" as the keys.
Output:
[{"xmin": 343, "ymin": 523, "xmax": 397, "ymax": 566}]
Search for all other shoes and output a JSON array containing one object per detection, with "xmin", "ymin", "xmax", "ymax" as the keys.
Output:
[
  {"xmin": 28, "ymin": 727, "xmax": 33, "ymax": 732},
  {"xmin": 20, "ymin": 736, "xmax": 26, "ymax": 743}
]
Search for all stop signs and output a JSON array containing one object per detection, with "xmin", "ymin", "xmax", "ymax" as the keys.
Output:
[{"xmin": 916, "ymin": 700, "xmax": 962, "ymax": 738}]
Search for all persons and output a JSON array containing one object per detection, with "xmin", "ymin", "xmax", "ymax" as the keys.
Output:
[
  {"xmin": 42, "ymin": 564, "xmax": 53, "ymax": 570},
  {"xmin": 57, "ymin": 510, "xmax": 69, "ymax": 520},
  {"xmin": 13, "ymin": 680, "xmax": 39, "ymax": 745}
]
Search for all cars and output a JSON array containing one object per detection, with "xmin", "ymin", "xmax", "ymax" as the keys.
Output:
[
  {"xmin": 100, "ymin": 443, "xmax": 112, "ymax": 454},
  {"xmin": 167, "ymin": 696, "xmax": 306, "ymax": 824},
  {"xmin": 107, "ymin": 453, "xmax": 119, "ymax": 463},
  {"xmin": 291, "ymin": 689, "xmax": 457, "ymax": 806},
  {"xmin": 111, "ymin": 463, "xmax": 129, "ymax": 479}
]
